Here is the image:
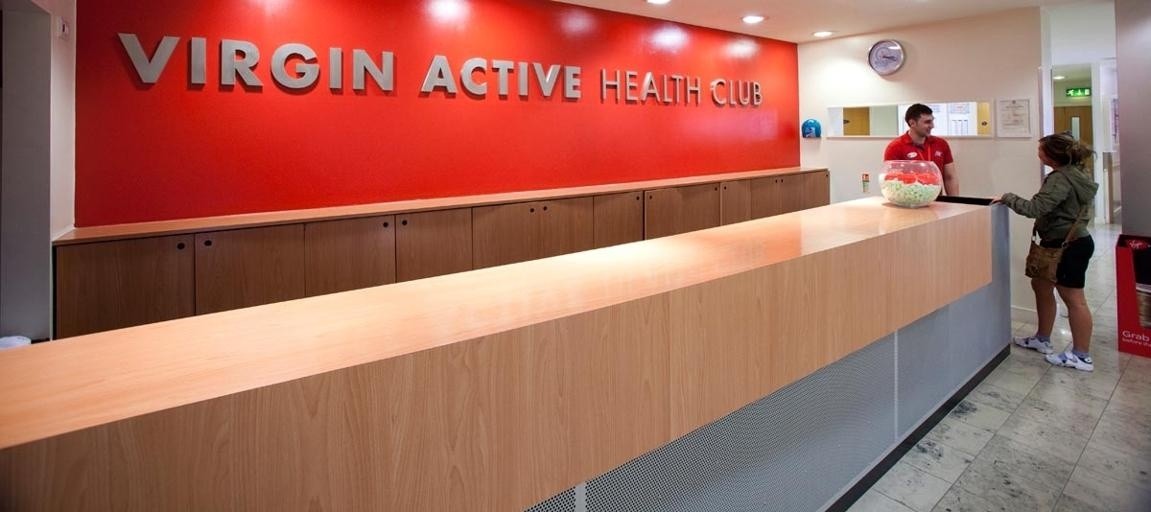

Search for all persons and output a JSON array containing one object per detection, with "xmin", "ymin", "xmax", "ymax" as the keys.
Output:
[
  {"xmin": 989, "ymin": 134, "xmax": 1099, "ymax": 372},
  {"xmin": 884, "ymin": 103, "xmax": 960, "ymax": 198}
]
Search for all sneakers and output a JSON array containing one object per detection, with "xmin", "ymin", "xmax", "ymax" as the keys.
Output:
[
  {"xmin": 1045, "ymin": 349, "xmax": 1095, "ymax": 372},
  {"xmin": 1014, "ymin": 333, "xmax": 1054, "ymax": 354}
]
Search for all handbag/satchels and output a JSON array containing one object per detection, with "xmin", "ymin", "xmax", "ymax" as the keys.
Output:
[{"xmin": 1024, "ymin": 240, "xmax": 1066, "ymax": 283}]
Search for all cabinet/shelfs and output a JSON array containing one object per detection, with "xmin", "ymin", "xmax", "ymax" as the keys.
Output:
[{"xmin": 53, "ymin": 164, "xmax": 832, "ymax": 341}]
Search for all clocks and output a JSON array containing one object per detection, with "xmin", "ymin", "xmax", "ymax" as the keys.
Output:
[{"xmin": 869, "ymin": 39, "xmax": 905, "ymax": 76}]
